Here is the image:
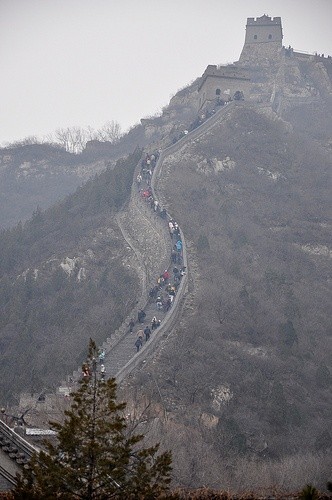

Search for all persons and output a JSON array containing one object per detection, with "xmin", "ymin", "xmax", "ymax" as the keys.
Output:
[
  {"xmin": 100, "ymin": 365, "xmax": 107, "ymax": 379},
  {"xmin": 82, "ymin": 367, "xmax": 91, "ymax": 378},
  {"xmin": 14, "ymin": 420, "xmax": 24, "ymax": 437},
  {"xmin": 99, "ymin": 347, "xmax": 106, "ymax": 363},
  {"xmin": 129, "ymin": 91, "xmax": 245, "ymax": 355},
  {"xmin": 64, "ymin": 395, "xmax": 70, "ymax": 401}
]
